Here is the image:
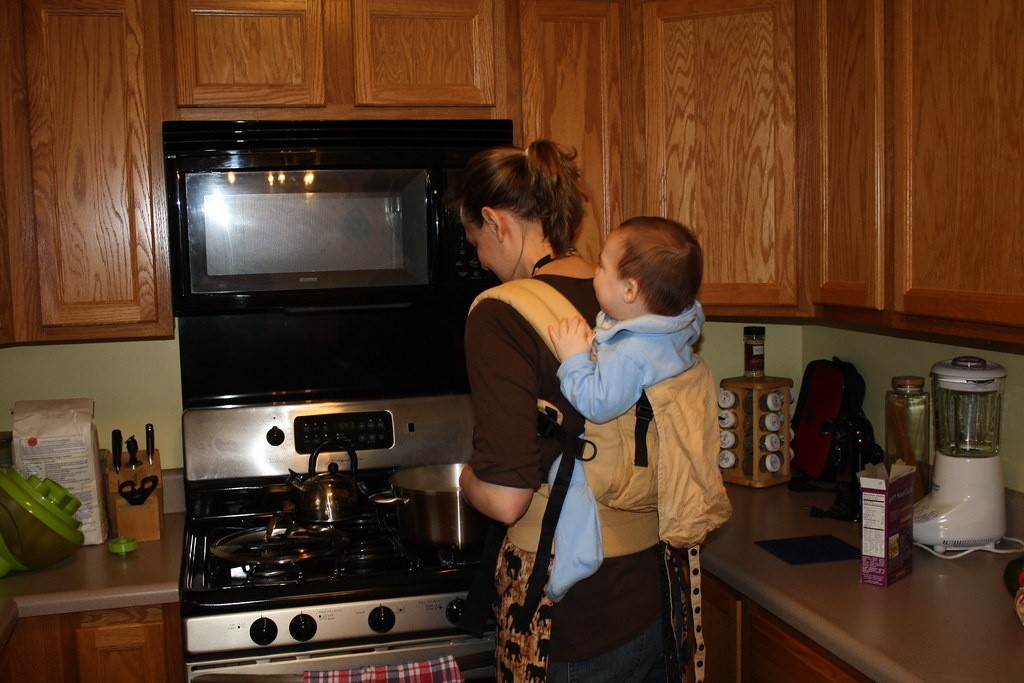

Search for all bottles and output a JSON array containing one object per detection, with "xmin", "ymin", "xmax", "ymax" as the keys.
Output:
[
  {"xmin": 719, "ymin": 325, "xmax": 795, "ymax": 488},
  {"xmin": 884, "ymin": 375, "xmax": 930, "ymax": 502}
]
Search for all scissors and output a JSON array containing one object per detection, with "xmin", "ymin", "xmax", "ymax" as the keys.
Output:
[{"xmin": 119, "ymin": 475, "xmax": 158, "ymax": 506}]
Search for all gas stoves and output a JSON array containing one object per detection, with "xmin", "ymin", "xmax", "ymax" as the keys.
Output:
[{"xmin": 180, "ymin": 395, "xmax": 506, "ymax": 653}]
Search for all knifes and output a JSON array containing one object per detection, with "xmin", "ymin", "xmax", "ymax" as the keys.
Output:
[{"xmin": 111, "ymin": 423, "xmax": 159, "ymax": 506}]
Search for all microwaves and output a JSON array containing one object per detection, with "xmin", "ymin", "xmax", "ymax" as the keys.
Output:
[{"xmin": 160, "ymin": 121, "xmax": 513, "ymax": 312}]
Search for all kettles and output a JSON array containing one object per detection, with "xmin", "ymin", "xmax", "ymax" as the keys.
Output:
[{"xmin": 284, "ymin": 438, "xmax": 364, "ymax": 523}]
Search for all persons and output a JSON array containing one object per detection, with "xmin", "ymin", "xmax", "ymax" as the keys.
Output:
[
  {"xmin": 456, "ymin": 139, "xmax": 696, "ymax": 683},
  {"xmin": 548, "ymin": 216, "xmax": 708, "ymax": 600}
]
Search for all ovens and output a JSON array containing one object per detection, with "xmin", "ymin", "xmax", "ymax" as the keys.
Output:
[{"xmin": 186, "ymin": 633, "xmax": 500, "ymax": 683}]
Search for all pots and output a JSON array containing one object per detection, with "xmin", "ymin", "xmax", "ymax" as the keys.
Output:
[{"xmin": 369, "ymin": 462, "xmax": 485, "ymax": 545}]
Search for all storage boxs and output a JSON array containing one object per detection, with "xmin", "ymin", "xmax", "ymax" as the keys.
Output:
[
  {"xmin": 856, "ymin": 459, "xmax": 919, "ymax": 588},
  {"xmin": 11, "ymin": 398, "xmax": 108, "ymax": 547}
]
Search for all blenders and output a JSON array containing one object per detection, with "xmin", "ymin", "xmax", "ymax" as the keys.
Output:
[{"xmin": 913, "ymin": 355, "xmax": 1024, "ymax": 559}]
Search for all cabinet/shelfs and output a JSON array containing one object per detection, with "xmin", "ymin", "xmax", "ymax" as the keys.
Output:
[
  {"xmin": 0, "ymin": 602, "xmax": 185, "ymax": 683},
  {"xmin": 679, "ymin": 560, "xmax": 876, "ymax": 683},
  {"xmin": 0, "ymin": 0, "xmax": 1024, "ymax": 354}
]
